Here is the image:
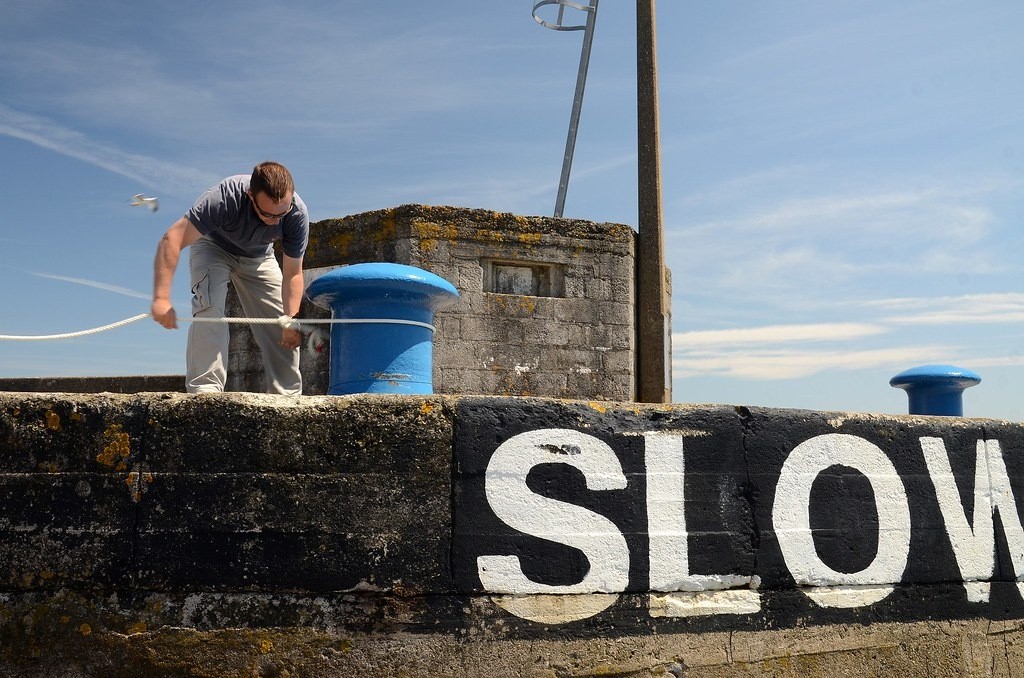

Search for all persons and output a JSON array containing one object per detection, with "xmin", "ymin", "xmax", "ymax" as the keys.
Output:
[{"xmin": 152, "ymin": 162, "xmax": 309, "ymax": 395}]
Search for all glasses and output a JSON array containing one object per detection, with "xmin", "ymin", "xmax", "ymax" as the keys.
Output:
[{"xmin": 253, "ymin": 196, "xmax": 288, "ymax": 219}]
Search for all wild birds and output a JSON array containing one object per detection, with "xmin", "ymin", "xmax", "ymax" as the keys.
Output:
[{"xmin": 126, "ymin": 192, "xmax": 158, "ymax": 212}]
